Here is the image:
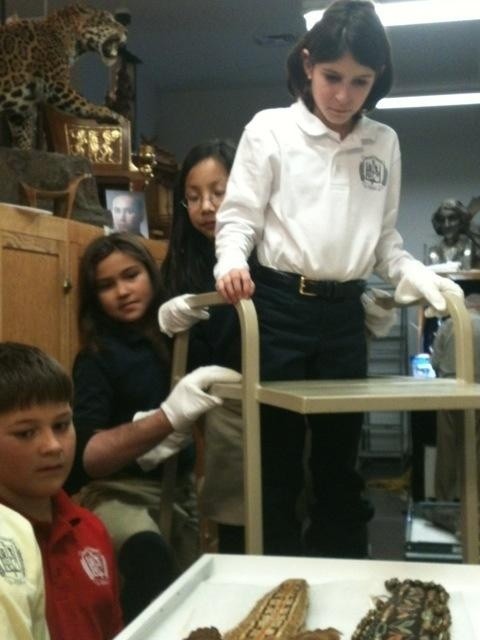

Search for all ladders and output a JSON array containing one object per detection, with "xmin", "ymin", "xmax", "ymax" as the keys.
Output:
[{"xmin": 354, "ymin": 282, "xmax": 416, "ymax": 475}]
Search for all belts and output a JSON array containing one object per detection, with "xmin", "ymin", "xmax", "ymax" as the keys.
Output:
[{"xmin": 250, "ymin": 263, "xmax": 368, "ymax": 302}]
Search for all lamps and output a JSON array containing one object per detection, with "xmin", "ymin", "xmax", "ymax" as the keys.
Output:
[
  {"xmin": 303, "ymin": 1, "xmax": 480, "ymax": 31},
  {"xmin": 376, "ymin": 92, "xmax": 480, "ymax": 110}
]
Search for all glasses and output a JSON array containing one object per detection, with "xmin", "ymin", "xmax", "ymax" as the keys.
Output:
[{"xmin": 178, "ymin": 187, "xmax": 229, "ymax": 209}]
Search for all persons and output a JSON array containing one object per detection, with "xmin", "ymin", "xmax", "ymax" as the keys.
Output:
[
  {"xmin": 214, "ymin": 1, "xmax": 466, "ymax": 560},
  {"xmin": 156, "ymin": 140, "xmax": 282, "ymax": 553},
  {"xmin": 432, "ymin": 294, "xmax": 480, "ymax": 504},
  {"xmin": 68, "ymin": 230, "xmax": 243, "ymax": 629},
  {"xmin": 0, "ymin": 340, "xmax": 126, "ymax": 640},
  {"xmin": 112, "ymin": 194, "xmax": 145, "ymax": 238},
  {"xmin": 427, "ymin": 199, "xmax": 474, "ymax": 272},
  {"xmin": 0, "ymin": 503, "xmax": 52, "ymax": 640}
]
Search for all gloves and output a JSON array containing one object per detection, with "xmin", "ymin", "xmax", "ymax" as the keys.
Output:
[
  {"xmin": 360, "ymin": 286, "xmax": 399, "ymax": 339},
  {"xmin": 129, "ymin": 408, "xmax": 190, "ymax": 472},
  {"xmin": 394, "ymin": 260, "xmax": 467, "ymax": 320},
  {"xmin": 157, "ymin": 362, "xmax": 244, "ymax": 431},
  {"xmin": 155, "ymin": 292, "xmax": 212, "ymax": 338}
]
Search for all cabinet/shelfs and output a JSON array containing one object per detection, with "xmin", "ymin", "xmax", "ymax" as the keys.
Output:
[{"xmin": 0, "ymin": 203, "xmax": 170, "ymax": 378}]
